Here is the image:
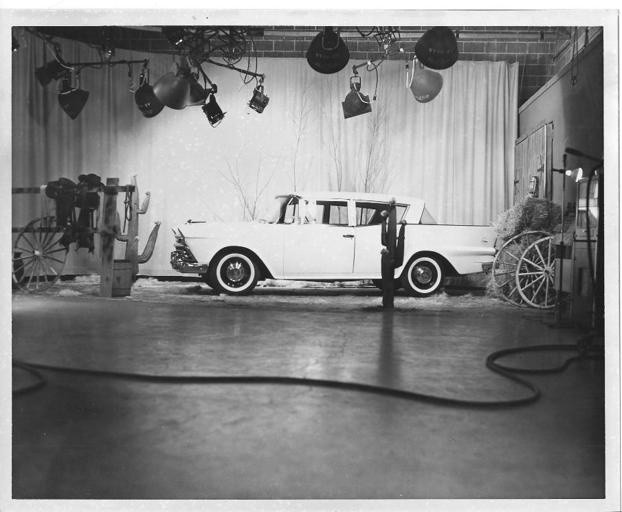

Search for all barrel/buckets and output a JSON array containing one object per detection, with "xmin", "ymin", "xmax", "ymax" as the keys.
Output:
[{"xmin": 112, "ymin": 258, "xmax": 132, "ymax": 297}]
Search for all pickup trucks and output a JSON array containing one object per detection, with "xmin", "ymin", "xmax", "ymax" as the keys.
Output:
[{"xmin": 170, "ymin": 191, "xmax": 500, "ymax": 298}]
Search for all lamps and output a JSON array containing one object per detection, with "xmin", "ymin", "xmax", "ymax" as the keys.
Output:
[
  {"xmin": 35, "ymin": 55, "xmax": 89, "ymax": 121},
  {"xmin": 306, "ymin": 25, "xmax": 459, "ymax": 118},
  {"xmin": 127, "ymin": 26, "xmax": 227, "ymax": 128},
  {"xmin": 249, "ymin": 84, "xmax": 270, "ymax": 113}
]
